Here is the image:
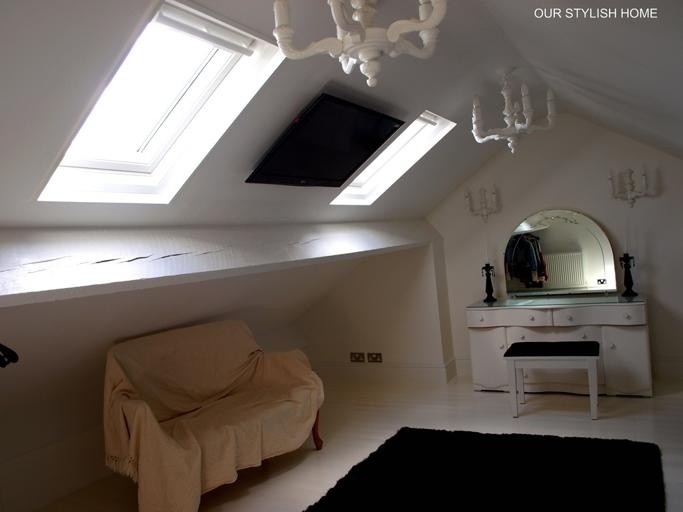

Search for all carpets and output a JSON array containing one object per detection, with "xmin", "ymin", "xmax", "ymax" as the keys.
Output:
[{"xmin": 301, "ymin": 426, "xmax": 666, "ymax": 512}]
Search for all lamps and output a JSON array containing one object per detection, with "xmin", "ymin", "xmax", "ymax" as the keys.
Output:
[
  {"xmin": 463, "ymin": 183, "xmax": 497, "ymax": 223},
  {"xmin": 271, "ymin": 1, "xmax": 449, "ymax": 88},
  {"xmin": 470, "ymin": 76, "xmax": 558, "ymax": 156},
  {"xmin": 605, "ymin": 164, "xmax": 648, "ymax": 208}
]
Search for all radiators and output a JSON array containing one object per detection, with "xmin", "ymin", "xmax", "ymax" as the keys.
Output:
[{"xmin": 539, "ymin": 252, "xmax": 585, "ymax": 288}]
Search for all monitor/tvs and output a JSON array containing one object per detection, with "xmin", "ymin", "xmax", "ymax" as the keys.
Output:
[{"xmin": 244, "ymin": 92, "xmax": 406, "ymax": 188}]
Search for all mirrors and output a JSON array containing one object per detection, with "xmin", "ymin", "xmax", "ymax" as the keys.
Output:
[{"xmin": 503, "ymin": 207, "xmax": 618, "ymax": 297}]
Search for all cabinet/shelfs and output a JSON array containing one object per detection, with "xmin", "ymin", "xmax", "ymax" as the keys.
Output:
[{"xmin": 464, "ymin": 292, "xmax": 654, "ymax": 398}]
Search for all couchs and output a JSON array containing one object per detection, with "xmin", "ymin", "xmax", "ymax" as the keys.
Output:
[{"xmin": 101, "ymin": 317, "xmax": 325, "ymax": 512}]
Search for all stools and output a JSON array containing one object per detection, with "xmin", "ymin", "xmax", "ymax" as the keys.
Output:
[{"xmin": 502, "ymin": 341, "xmax": 601, "ymax": 419}]
